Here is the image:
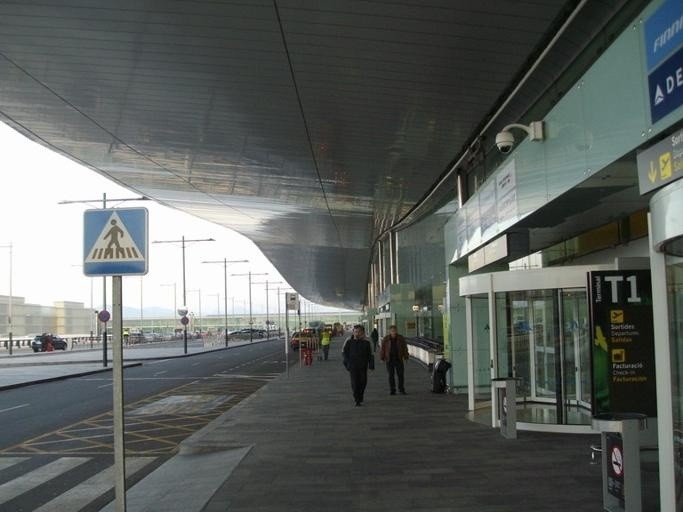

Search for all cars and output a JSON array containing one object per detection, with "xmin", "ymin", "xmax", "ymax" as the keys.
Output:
[
  {"xmin": 291, "ymin": 328, "xmax": 319, "ymax": 350},
  {"xmin": 114, "ymin": 323, "xmax": 282, "ymax": 347}
]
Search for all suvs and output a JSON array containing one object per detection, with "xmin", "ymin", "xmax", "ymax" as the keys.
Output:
[{"xmin": 31, "ymin": 333, "xmax": 67, "ymax": 353}]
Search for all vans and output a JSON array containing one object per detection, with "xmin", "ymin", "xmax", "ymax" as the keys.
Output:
[{"xmin": 306, "ymin": 321, "xmax": 342, "ymax": 338}]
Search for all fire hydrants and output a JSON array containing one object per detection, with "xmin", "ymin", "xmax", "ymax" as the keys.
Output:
[{"xmin": 303, "ymin": 350, "xmax": 312, "ymax": 367}]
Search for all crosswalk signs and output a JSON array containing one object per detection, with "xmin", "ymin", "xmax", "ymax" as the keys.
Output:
[{"xmin": 81, "ymin": 208, "xmax": 148, "ymax": 278}]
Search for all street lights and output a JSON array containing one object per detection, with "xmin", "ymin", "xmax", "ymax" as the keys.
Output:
[
  {"xmin": 151, "ymin": 237, "xmax": 215, "ymax": 356},
  {"xmin": 227, "ymin": 272, "xmax": 315, "ymax": 339},
  {"xmin": 62, "ymin": 195, "xmax": 152, "ymax": 367},
  {"xmin": 201, "ymin": 258, "xmax": 247, "ymax": 348}
]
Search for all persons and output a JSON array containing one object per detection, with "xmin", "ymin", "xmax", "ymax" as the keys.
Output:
[{"xmin": 318, "ymin": 322, "xmax": 410, "ymax": 407}]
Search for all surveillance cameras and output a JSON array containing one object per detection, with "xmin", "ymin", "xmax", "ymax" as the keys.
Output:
[{"xmin": 494, "ymin": 131, "xmax": 515, "ymax": 154}]
